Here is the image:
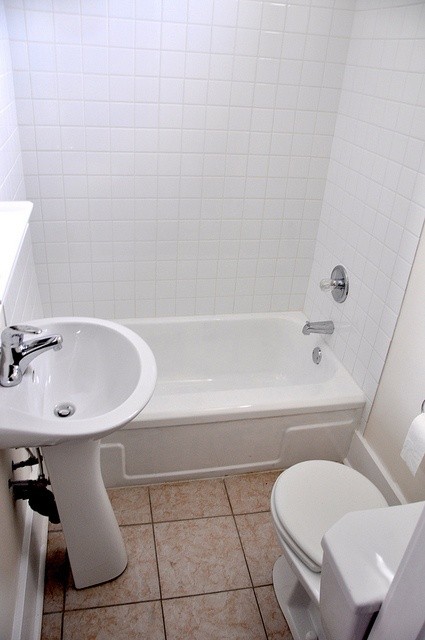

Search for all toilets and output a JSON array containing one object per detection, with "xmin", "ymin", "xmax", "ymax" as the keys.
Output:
[{"xmin": 271, "ymin": 458, "xmax": 425, "ymax": 639}]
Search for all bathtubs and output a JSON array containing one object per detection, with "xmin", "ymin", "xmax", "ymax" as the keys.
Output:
[{"xmin": 93, "ymin": 311, "xmax": 364, "ymax": 488}]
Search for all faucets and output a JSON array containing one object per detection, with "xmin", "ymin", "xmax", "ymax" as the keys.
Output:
[{"xmin": 0, "ymin": 323, "xmax": 63, "ymax": 389}]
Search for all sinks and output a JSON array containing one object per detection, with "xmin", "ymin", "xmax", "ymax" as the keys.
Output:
[{"xmin": 21, "ymin": 322, "xmax": 142, "ymax": 423}]
